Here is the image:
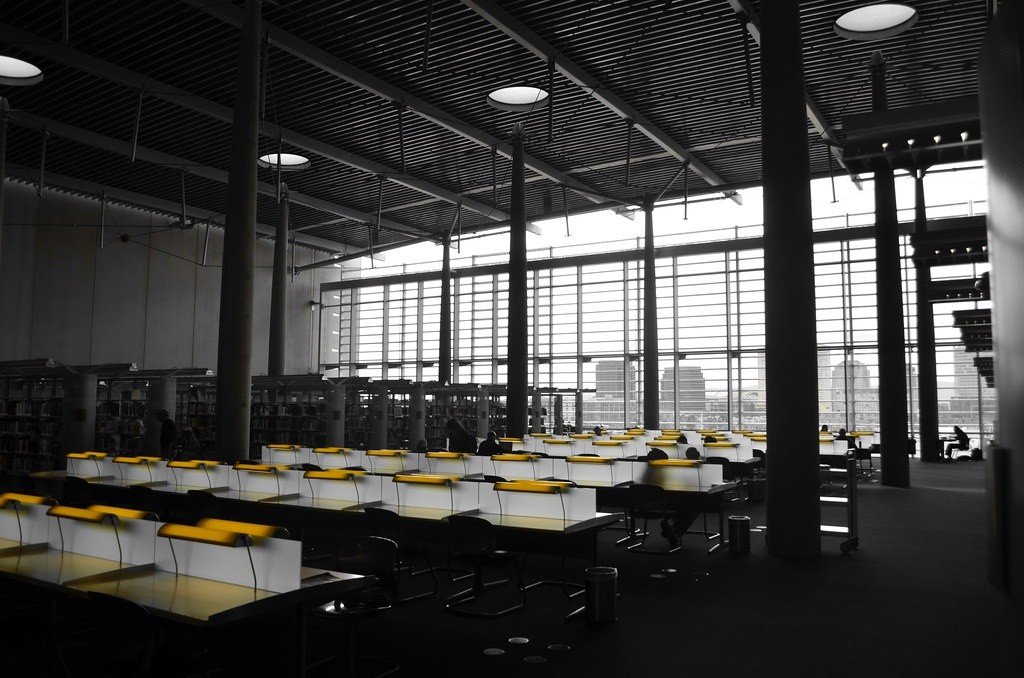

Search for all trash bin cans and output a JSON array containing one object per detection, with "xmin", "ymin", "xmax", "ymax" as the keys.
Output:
[
  {"xmin": 584, "ymin": 567, "xmax": 618, "ymax": 623},
  {"xmin": 728, "ymin": 515, "xmax": 751, "ymax": 553}
]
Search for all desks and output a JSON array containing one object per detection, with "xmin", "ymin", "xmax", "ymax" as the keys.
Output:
[{"xmin": 0, "ymin": 425, "xmax": 983, "ymax": 678}]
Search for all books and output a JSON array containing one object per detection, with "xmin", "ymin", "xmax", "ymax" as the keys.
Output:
[
  {"xmin": 450, "ymin": 401, "xmax": 477, "ymax": 433},
  {"xmin": 0, "ymin": 399, "xmax": 64, "ymax": 416},
  {"xmin": 94, "ymin": 434, "xmax": 146, "ymax": 451},
  {"xmin": 172, "ymin": 415, "xmax": 214, "ymax": 428},
  {"xmin": 249, "ymin": 431, "xmax": 325, "ymax": 448},
  {"xmin": 192, "ymin": 430, "xmax": 217, "ymax": 441},
  {"xmin": 249, "ymin": 417, "xmax": 325, "ymax": 431},
  {"xmin": 93, "ymin": 398, "xmax": 148, "ymax": 417},
  {"xmin": 345, "ymin": 401, "xmax": 372, "ymax": 448},
  {"xmin": 0, "ymin": 437, "xmax": 61, "ymax": 453},
  {"xmin": 1, "ymin": 452, "xmax": 58, "ymax": 474},
  {"xmin": 247, "ymin": 403, "xmax": 329, "ymax": 417},
  {"xmin": 96, "ymin": 418, "xmax": 146, "ymax": 434},
  {"xmin": 0, "ymin": 418, "xmax": 63, "ymax": 436},
  {"xmin": 248, "ymin": 443, "xmax": 261, "ymax": 460},
  {"xmin": 179, "ymin": 401, "xmax": 214, "ymax": 414},
  {"xmin": 424, "ymin": 404, "xmax": 448, "ymax": 449},
  {"xmin": 388, "ymin": 402, "xmax": 412, "ymax": 450}
]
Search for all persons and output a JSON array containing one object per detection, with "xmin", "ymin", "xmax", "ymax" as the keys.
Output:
[
  {"xmin": 669, "ymin": 447, "xmax": 721, "ymax": 547},
  {"xmin": 834, "ymin": 428, "xmax": 858, "ymax": 450},
  {"xmin": 478, "ymin": 430, "xmax": 527, "ymax": 458},
  {"xmin": 445, "ymin": 417, "xmax": 478, "ymax": 454},
  {"xmin": 703, "ymin": 436, "xmax": 717, "ymax": 444},
  {"xmin": 943, "ymin": 425, "xmax": 969, "ymax": 461},
  {"xmin": 157, "ymin": 405, "xmax": 177, "ymax": 460},
  {"xmin": 821, "ymin": 424, "xmax": 829, "ymax": 432},
  {"xmin": 628, "ymin": 449, "xmax": 678, "ymax": 546},
  {"xmin": 415, "ymin": 438, "xmax": 430, "ymax": 454},
  {"xmin": 677, "ymin": 435, "xmax": 687, "ymax": 444},
  {"xmin": 173, "ymin": 424, "xmax": 202, "ymax": 460},
  {"xmin": 594, "ymin": 426, "xmax": 604, "ymax": 436}
]
